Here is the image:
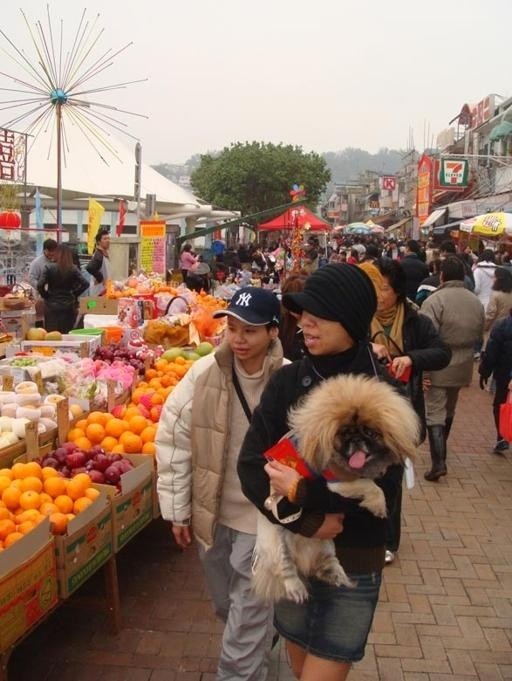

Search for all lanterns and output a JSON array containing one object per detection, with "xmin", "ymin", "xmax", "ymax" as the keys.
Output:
[{"xmin": 0, "ymin": 208, "xmax": 21, "ymax": 234}]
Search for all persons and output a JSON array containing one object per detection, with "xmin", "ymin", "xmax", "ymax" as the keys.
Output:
[
  {"xmin": 155, "ymin": 287, "xmax": 293, "ymax": 680},
  {"xmin": 420, "ymin": 262, "xmax": 486, "ymax": 480},
  {"xmin": 300, "ymin": 231, "xmax": 512, "ymax": 392},
  {"xmin": 478, "ymin": 310, "xmax": 512, "ymax": 451},
  {"xmin": 27, "ymin": 239, "xmax": 89, "ymax": 334},
  {"xmin": 369, "ymin": 260, "xmax": 451, "ymax": 562},
  {"xmin": 179, "ymin": 232, "xmax": 295, "ymax": 291},
  {"xmin": 87, "ymin": 233, "xmax": 116, "ymax": 283},
  {"xmin": 236, "ymin": 261, "xmax": 403, "ymax": 679}
]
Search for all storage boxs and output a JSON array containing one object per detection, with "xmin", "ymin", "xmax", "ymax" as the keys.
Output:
[
  {"xmin": 11, "ymin": 442, "xmax": 157, "ymax": 553},
  {"xmin": 2, "ymin": 373, "xmax": 145, "ymax": 466},
  {"xmin": 1, "ymin": 298, "xmax": 103, "ymax": 364},
  {"xmin": 59, "ymin": 482, "xmax": 115, "ymax": 601},
  {"xmin": 78, "ymin": 290, "xmax": 121, "ymax": 317},
  {"xmin": 1, "ymin": 516, "xmax": 59, "ymax": 658}
]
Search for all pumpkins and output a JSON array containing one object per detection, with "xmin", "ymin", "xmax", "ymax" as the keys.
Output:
[{"xmin": 0, "ymin": 212, "xmax": 21, "ymax": 227}]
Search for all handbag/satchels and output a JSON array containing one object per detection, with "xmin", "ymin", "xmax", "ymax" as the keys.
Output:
[
  {"xmin": 372, "ymin": 330, "xmax": 413, "ymax": 385},
  {"xmin": 498, "ymin": 403, "xmax": 512, "ymax": 443}
]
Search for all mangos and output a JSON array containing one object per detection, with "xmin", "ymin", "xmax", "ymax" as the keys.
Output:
[{"xmin": 26, "ymin": 327, "xmax": 62, "ymax": 340}]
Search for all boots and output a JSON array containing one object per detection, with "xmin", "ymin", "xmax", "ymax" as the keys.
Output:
[{"xmin": 424, "ymin": 415, "xmax": 454, "ymax": 481}]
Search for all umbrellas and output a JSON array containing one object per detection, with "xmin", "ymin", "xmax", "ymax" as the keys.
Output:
[{"xmin": 458, "ymin": 212, "xmax": 512, "ymax": 236}]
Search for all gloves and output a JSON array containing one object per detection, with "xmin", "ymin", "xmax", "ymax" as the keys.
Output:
[{"xmin": 479, "ymin": 375, "xmax": 488, "ymax": 390}]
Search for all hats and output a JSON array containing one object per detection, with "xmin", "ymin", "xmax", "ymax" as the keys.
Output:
[
  {"xmin": 281, "ymin": 262, "xmax": 378, "ymax": 344},
  {"xmin": 186, "ymin": 261, "xmax": 211, "ymax": 278},
  {"xmin": 213, "ymin": 287, "xmax": 280, "ymax": 327},
  {"xmin": 212, "ymin": 240, "xmax": 225, "ymax": 256}
]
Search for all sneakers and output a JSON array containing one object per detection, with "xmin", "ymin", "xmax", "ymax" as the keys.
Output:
[{"xmin": 493, "ymin": 441, "xmax": 509, "ymax": 455}]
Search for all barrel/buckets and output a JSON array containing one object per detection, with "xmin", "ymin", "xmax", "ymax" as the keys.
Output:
[{"xmin": 237, "ymin": 268, "xmax": 252, "ymax": 286}]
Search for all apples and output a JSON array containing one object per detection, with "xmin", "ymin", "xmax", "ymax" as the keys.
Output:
[
  {"xmin": 93, "ymin": 343, "xmax": 154, "ymax": 375},
  {"xmin": 0, "ymin": 356, "xmax": 193, "ymax": 454}
]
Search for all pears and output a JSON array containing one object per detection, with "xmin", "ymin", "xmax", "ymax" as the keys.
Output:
[{"xmin": 162, "ymin": 340, "xmax": 213, "ymax": 361}]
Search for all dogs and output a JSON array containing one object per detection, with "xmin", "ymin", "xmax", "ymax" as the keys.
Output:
[{"xmin": 249, "ymin": 373, "xmax": 423, "ymax": 609}]
